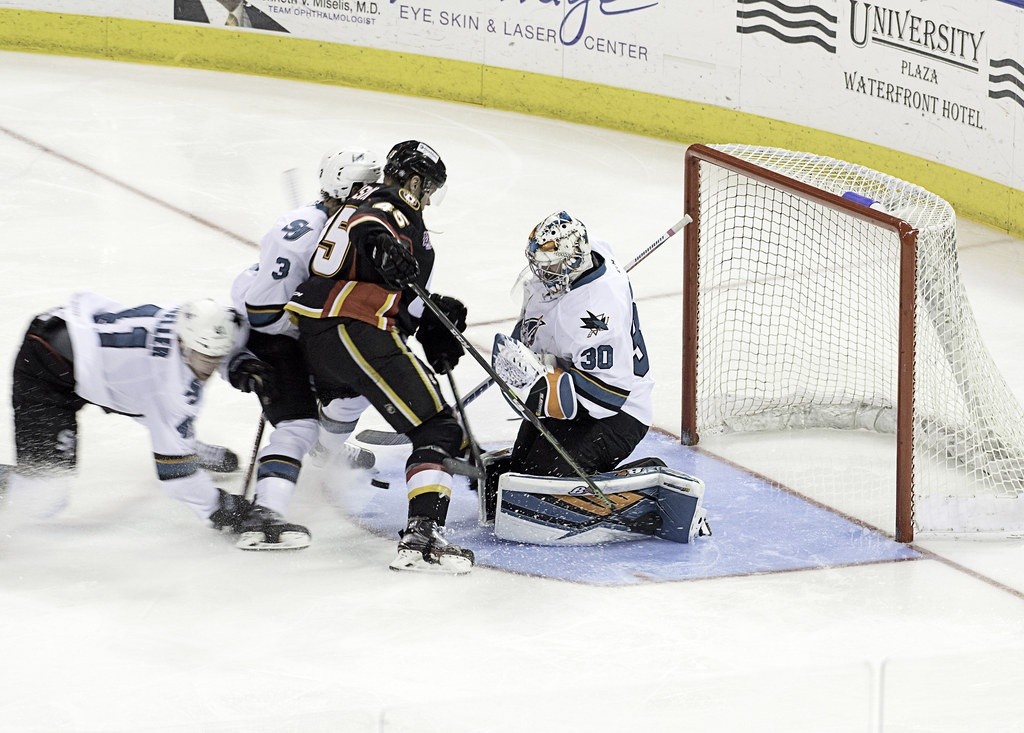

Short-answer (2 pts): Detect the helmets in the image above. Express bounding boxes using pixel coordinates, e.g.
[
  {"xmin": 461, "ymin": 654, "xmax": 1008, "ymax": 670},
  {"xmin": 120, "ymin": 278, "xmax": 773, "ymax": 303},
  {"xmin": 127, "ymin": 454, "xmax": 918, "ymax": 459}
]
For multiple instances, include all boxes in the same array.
[
  {"xmin": 179, "ymin": 297, "xmax": 249, "ymax": 358},
  {"xmin": 525, "ymin": 212, "xmax": 590, "ymax": 292},
  {"xmin": 384, "ymin": 141, "xmax": 448, "ymax": 191},
  {"xmin": 319, "ymin": 148, "xmax": 382, "ymax": 203}
]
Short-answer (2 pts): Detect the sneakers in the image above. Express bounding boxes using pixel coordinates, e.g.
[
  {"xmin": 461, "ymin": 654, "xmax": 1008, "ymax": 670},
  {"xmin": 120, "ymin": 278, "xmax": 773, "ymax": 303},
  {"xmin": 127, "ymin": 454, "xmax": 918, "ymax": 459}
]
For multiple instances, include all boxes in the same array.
[
  {"xmin": 193, "ymin": 439, "xmax": 240, "ymax": 475},
  {"xmin": 238, "ymin": 503, "xmax": 311, "ymax": 552},
  {"xmin": 389, "ymin": 521, "xmax": 474, "ymax": 572},
  {"xmin": 309, "ymin": 445, "xmax": 376, "ymax": 471}
]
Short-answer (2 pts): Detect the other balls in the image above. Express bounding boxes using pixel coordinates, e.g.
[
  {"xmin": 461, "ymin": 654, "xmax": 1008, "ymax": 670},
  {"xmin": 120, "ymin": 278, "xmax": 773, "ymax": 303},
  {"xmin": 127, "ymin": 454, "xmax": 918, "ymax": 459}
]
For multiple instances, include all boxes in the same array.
[{"xmin": 371, "ymin": 478, "xmax": 391, "ymax": 490}]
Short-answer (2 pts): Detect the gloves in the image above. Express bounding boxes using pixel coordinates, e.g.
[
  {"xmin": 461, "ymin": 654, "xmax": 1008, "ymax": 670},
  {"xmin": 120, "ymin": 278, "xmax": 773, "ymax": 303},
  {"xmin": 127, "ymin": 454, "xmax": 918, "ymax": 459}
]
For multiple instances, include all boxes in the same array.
[
  {"xmin": 423, "ymin": 290, "xmax": 467, "ymax": 336},
  {"xmin": 416, "ymin": 324, "xmax": 465, "ymax": 375},
  {"xmin": 210, "ymin": 489, "xmax": 250, "ymax": 529}
]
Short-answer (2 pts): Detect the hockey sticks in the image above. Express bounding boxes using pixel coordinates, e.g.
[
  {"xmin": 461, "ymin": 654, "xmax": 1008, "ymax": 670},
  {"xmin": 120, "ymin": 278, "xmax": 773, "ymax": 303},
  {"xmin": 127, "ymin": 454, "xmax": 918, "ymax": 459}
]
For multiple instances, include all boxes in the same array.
[
  {"xmin": 354, "ymin": 212, "xmax": 695, "ymax": 447},
  {"xmin": 441, "ymin": 357, "xmax": 488, "ymax": 482},
  {"xmin": 405, "ymin": 281, "xmax": 666, "ymax": 536},
  {"xmin": 209, "ymin": 408, "xmax": 266, "ymax": 528}
]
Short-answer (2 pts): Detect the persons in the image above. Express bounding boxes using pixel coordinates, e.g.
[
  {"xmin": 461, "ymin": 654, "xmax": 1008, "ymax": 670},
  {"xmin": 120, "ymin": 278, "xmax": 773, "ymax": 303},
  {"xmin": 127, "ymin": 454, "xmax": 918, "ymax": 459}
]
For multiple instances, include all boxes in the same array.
[
  {"xmin": 231, "ymin": 141, "xmax": 476, "ymax": 559},
  {"xmin": 0, "ymin": 290, "xmax": 252, "ymax": 534},
  {"xmin": 482, "ymin": 211, "xmax": 713, "ymax": 547}
]
